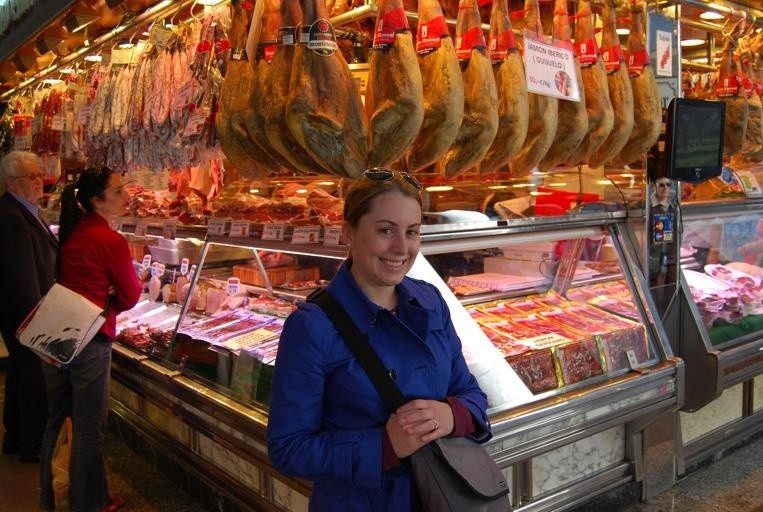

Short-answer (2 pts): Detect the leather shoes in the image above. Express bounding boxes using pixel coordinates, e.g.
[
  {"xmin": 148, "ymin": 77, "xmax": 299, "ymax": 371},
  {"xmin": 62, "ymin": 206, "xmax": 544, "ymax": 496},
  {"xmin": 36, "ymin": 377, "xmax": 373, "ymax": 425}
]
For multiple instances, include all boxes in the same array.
[{"xmin": 98, "ymin": 494, "xmax": 125, "ymax": 512}]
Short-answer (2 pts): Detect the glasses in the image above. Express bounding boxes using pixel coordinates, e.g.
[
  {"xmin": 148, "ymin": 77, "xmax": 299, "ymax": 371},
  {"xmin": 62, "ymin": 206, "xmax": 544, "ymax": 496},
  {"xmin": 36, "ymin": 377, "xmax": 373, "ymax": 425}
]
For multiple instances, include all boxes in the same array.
[
  {"xmin": 8, "ymin": 174, "xmax": 43, "ymax": 180},
  {"xmin": 95, "ymin": 166, "xmax": 110, "ymax": 182},
  {"xmin": 659, "ymin": 183, "xmax": 670, "ymax": 187},
  {"xmin": 358, "ymin": 168, "xmax": 425, "ymax": 193}
]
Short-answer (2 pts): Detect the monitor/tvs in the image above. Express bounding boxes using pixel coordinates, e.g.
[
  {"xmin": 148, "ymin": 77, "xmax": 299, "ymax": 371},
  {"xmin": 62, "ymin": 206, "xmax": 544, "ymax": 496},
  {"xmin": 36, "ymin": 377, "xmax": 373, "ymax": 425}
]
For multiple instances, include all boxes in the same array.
[{"xmin": 664, "ymin": 97, "xmax": 726, "ymax": 181}]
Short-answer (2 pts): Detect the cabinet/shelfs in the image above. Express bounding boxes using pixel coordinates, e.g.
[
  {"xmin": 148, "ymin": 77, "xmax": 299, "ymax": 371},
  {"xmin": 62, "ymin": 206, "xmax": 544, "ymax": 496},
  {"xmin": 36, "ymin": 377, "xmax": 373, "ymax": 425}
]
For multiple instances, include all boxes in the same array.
[{"xmin": 39, "ymin": 194, "xmax": 762, "ymax": 511}]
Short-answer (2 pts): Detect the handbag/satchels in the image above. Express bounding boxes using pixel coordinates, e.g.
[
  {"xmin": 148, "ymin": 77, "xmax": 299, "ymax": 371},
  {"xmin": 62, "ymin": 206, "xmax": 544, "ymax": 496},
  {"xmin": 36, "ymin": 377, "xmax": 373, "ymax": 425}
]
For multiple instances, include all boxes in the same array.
[
  {"xmin": 16, "ymin": 281, "xmax": 109, "ymax": 371},
  {"xmin": 407, "ymin": 434, "xmax": 511, "ymax": 510}
]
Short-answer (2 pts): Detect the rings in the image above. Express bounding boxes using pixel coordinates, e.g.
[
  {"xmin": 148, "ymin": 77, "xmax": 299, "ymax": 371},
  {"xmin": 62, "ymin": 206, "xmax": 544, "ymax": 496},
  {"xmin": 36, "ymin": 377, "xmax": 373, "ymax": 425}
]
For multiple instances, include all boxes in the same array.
[{"xmin": 432, "ymin": 420, "xmax": 437, "ymax": 429}]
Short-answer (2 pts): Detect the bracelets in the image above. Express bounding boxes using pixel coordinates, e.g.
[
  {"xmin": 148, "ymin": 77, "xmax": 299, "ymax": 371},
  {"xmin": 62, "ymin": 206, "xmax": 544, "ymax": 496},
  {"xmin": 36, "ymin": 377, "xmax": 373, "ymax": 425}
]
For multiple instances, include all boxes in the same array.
[{"xmin": 709, "ymin": 248, "xmax": 720, "ymax": 254}]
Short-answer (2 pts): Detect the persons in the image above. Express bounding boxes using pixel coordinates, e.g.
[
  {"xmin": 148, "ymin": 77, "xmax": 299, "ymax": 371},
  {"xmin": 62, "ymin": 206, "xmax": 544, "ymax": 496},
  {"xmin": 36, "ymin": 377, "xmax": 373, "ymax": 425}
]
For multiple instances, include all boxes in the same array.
[
  {"xmin": 30, "ymin": 166, "xmax": 143, "ymax": 510},
  {"xmin": 557, "ymin": 71, "xmax": 571, "ymax": 96},
  {"xmin": 650, "ymin": 178, "xmax": 676, "ymax": 210},
  {"xmin": 706, "ymin": 212, "xmax": 763, "ymax": 269},
  {"xmin": 265, "ymin": 169, "xmax": 493, "ymax": 512},
  {"xmin": 258, "ymin": 249, "xmax": 337, "ymax": 280},
  {"xmin": 680, "ymin": 217, "xmax": 725, "ymax": 274},
  {"xmin": 0, "ymin": 151, "xmax": 61, "ymax": 466}
]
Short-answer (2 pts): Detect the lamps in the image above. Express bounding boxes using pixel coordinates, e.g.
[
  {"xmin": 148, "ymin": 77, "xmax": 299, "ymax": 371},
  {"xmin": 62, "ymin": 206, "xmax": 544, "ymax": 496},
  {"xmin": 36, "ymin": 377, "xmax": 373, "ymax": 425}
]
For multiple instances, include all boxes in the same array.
[{"xmin": 680, "ymin": 24, "xmax": 709, "ymax": 47}]
